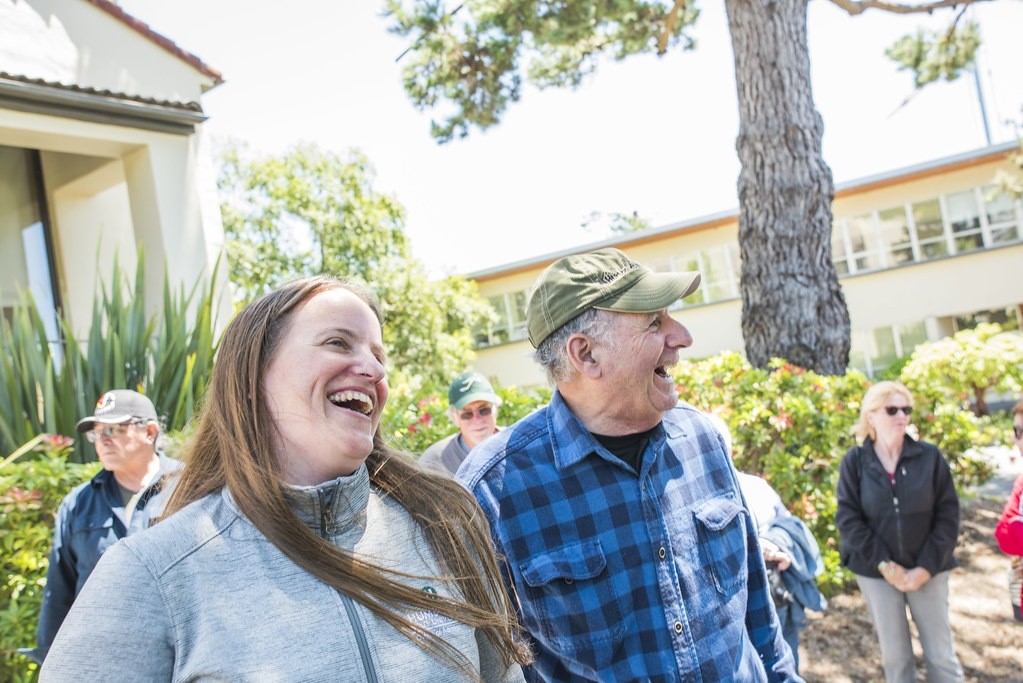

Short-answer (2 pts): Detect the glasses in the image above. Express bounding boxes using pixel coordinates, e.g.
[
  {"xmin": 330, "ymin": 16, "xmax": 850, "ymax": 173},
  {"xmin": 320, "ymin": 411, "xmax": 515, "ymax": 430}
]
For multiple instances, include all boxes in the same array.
[
  {"xmin": 457, "ymin": 404, "xmax": 491, "ymax": 420},
  {"xmin": 84, "ymin": 419, "xmax": 144, "ymax": 443},
  {"xmin": 884, "ymin": 405, "xmax": 913, "ymax": 416},
  {"xmin": 1013, "ymin": 425, "xmax": 1023, "ymax": 439}
]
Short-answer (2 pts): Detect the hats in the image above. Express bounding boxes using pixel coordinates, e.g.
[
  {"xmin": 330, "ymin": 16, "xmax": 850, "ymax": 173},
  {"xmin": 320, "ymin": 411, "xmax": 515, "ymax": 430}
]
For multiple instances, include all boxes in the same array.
[
  {"xmin": 449, "ymin": 372, "xmax": 499, "ymax": 409},
  {"xmin": 74, "ymin": 390, "xmax": 157, "ymax": 433},
  {"xmin": 526, "ymin": 247, "xmax": 701, "ymax": 348}
]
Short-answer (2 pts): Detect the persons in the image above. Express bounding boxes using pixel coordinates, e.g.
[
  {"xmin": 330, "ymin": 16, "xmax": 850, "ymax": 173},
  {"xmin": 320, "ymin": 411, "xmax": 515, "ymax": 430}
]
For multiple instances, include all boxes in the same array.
[
  {"xmin": 17, "ymin": 388, "xmax": 185, "ymax": 666},
  {"xmin": 453, "ymin": 248, "xmax": 805, "ymax": 683},
  {"xmin": 417, "ymin": 371, "xmax": 506, "ymax": 476},
  {"xmin": 39, "ymin": 275, "xmax": 529, "ymax": 683},
  {"xmin": 994, "ymin": 402, "xmax": 1023, "ymax": 618},
  {"xmin": 705, "ymin": 411, "xmax": 799, "ymax": 675},
  {"xmin": 832, "ymin": 381, "xmax": 964, "ymax": 683}
]
